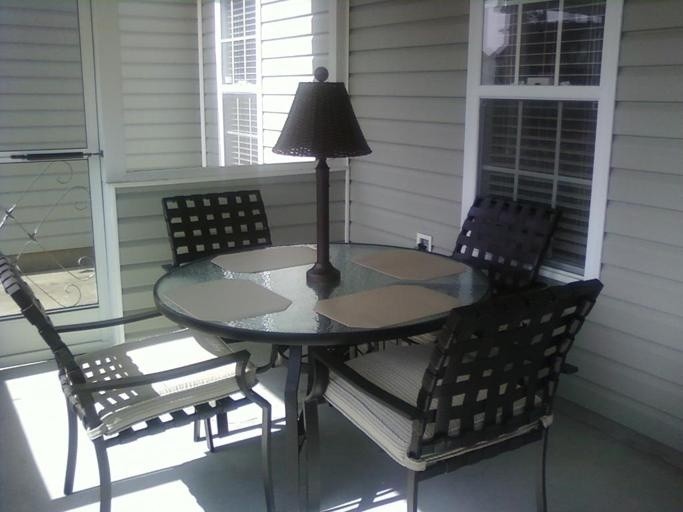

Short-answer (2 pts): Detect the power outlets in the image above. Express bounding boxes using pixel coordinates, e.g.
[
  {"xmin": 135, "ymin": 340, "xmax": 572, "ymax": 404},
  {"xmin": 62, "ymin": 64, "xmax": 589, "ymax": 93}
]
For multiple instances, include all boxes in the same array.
[{"xmin": 415, "ymin": 232, "xmax": 432, "ymax": 252}]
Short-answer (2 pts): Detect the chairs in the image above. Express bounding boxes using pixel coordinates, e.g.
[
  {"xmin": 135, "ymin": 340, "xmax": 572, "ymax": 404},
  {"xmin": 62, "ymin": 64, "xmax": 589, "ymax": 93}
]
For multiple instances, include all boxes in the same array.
[
  {"xmin": 289, "ymin": 275, "xmax": 605, "ymax": 512},
  {"xmin": 153, "ymin": 186, "xmax": 314, "ymax": 439},
  {"xmin": 328, "ymin": 191, "xmax": 567, "ymax": 365},
  {"xmin": 0, "ymin": 246, "xmax": 288, "ymax": 511}
]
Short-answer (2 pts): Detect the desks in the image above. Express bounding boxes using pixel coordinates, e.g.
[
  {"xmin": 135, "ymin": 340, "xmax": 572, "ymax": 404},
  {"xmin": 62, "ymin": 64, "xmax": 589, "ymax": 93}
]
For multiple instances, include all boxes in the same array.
[{"xmin": 147, "ymin": 238, "xmax": 506, "ymax": 511}]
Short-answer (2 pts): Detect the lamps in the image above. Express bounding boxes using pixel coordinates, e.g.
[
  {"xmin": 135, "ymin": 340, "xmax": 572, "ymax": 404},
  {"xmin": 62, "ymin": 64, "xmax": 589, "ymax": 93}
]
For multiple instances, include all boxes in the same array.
[{"xmin": 267, "ymin": 65, "xmax": 373, "ymax": 292}]
